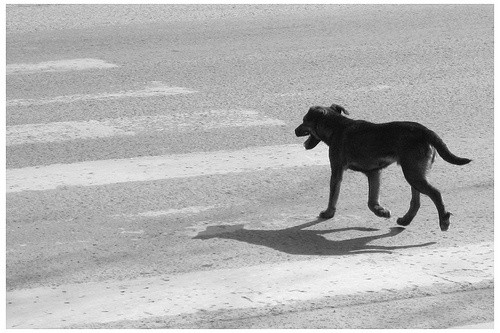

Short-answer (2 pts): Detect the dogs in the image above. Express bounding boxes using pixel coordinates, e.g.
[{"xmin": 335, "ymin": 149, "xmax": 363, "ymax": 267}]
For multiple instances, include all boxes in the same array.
[{"xmin": 294, "ymin": 102, "xmax": 475, "ymax": 231}]
